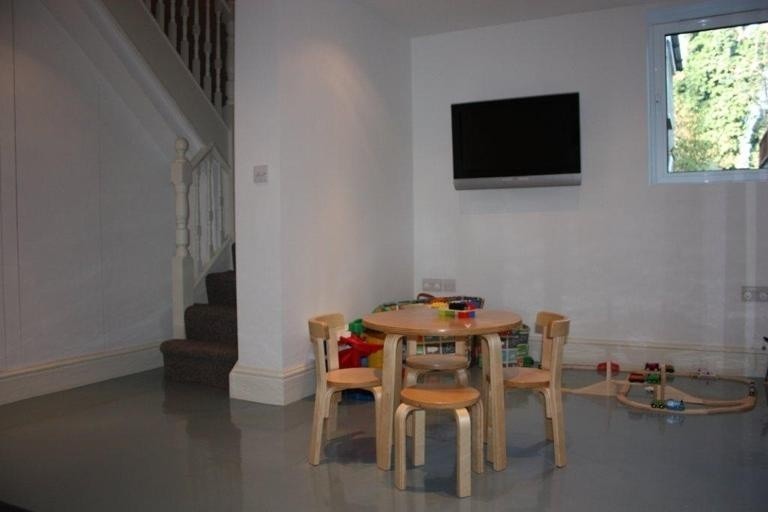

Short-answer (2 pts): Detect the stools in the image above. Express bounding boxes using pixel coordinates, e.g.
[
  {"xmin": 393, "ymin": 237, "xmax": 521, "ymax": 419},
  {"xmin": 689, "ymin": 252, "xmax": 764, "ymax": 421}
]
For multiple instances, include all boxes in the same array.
[
  {"xmin": 394, "ymin": 383, "xmax": 485, "ymax": 497},
  {"xmin": 403, "ymin": 353, "xmax": 471, "ymax": 438}
]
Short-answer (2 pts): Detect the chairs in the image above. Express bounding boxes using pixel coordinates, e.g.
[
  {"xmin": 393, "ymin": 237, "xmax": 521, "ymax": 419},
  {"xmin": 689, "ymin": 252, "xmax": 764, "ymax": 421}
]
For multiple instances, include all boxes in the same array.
[
  {"xmin": 486, "ymin": 311, "xmax": 570, "ymax": 468},
  {"xmin": 308, "ymin": 313, "xmax": 385, "ymax": 465}
]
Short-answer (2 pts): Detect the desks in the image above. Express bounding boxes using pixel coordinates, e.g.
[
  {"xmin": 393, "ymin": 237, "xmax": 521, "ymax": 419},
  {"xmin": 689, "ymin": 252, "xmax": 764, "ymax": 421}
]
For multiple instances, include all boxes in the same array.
[{"xmin": 362, "ymin": 308, "xmax": 523, "ymax": 471}]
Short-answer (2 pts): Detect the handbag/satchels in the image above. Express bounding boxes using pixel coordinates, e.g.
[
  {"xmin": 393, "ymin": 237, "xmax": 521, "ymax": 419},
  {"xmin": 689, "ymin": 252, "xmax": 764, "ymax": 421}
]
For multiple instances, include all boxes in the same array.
[
  {"xmin": 374, "ymin": 293, "xmax": 484, "ymax": 373},
  {"xmin": 476, "ymin": 324, "xmax": 529, "ymax": 368}
]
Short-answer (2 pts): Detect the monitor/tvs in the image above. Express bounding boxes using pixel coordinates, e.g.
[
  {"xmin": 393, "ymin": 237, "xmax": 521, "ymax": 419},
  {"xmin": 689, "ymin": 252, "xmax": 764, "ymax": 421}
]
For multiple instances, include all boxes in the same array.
[{"xmin": 450, "ymin": 91, "xmax": 582, "ymax": 190}]
